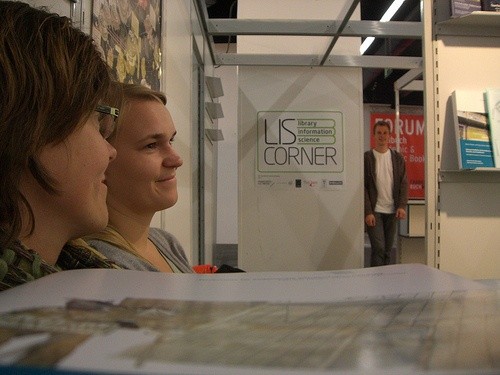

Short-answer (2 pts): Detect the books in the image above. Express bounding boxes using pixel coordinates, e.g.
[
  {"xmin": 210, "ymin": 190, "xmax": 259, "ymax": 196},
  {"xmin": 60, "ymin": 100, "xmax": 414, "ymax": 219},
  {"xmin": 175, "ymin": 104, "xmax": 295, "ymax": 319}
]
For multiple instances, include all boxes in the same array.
[
  {"xmin": 450, "ymin": 0, "xmax": 500, "ymax": 18},
  {"xmin": 450, "ymin": 88, "xmax": 500, "ymax": 171}
]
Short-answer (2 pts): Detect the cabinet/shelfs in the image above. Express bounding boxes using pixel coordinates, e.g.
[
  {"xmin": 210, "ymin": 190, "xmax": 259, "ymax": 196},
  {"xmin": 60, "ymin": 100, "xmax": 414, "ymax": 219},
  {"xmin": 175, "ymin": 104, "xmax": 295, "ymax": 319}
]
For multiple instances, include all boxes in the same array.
[
  {"xmin": 204, "ymin": 76, "xmax": 224, "ymax": 146},
  {"xmin": 423, "ymin": 0, "xmax": 500, "ymax": 279}
]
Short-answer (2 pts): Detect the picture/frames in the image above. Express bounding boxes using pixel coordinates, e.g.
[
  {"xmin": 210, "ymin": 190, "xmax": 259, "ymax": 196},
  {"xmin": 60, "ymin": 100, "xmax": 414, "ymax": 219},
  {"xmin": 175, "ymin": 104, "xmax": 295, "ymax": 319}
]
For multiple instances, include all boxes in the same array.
[{"xmin": 90, "ymin": 0, "xmax": 166, "ymax": 104}]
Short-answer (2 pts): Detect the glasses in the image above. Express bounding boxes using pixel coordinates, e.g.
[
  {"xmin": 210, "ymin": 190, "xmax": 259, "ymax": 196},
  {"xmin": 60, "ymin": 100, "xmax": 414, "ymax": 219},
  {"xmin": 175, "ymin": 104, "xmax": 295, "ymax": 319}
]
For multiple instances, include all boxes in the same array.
[{"xmin": 92, "ymin": 104, "xmax": 120, "ymax": 140}]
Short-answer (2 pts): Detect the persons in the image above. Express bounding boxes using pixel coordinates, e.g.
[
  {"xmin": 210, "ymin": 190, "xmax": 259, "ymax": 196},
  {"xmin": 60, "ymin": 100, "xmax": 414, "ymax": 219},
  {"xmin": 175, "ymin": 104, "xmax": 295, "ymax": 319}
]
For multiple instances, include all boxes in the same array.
[
  {"xmin": 0, "ymin": 0, "xmax": 121, "ymax": 294},
  {"xmin": 365, "ymin": 121, "xmax": 408, "ymax": 266},
  {"xmin": 84, "ymin": 83, "xmax": 200, "ymax": 273}
]
